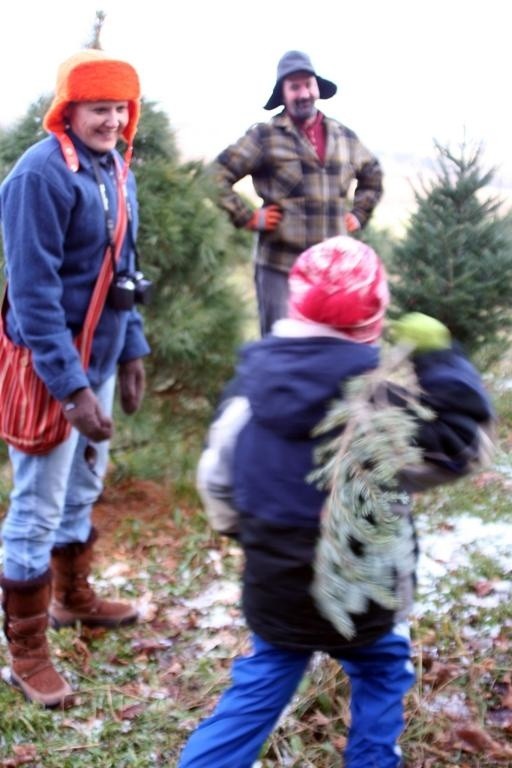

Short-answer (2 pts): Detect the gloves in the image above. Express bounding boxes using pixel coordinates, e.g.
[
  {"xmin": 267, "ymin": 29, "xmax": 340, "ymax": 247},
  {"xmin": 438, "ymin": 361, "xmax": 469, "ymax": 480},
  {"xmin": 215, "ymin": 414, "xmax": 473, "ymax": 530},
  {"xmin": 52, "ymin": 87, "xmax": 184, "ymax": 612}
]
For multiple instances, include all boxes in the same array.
[
  {"xmin": 244, "ymin": 205, "xmax": 282, "ymax": 231},
  {"xmin": 60, "ymin": 386, "xmax": 112, "ymax": 444},
  {"xmin": 343, "ymin": 212, "xmax": 362, "ymax": 233},
  {"xmin": 389, "ymin": 311, "xmax": 452, "ymax": 355},
  {"xmin": 119, "ymin": 358, "xmax": 147, "ymax": 415}
]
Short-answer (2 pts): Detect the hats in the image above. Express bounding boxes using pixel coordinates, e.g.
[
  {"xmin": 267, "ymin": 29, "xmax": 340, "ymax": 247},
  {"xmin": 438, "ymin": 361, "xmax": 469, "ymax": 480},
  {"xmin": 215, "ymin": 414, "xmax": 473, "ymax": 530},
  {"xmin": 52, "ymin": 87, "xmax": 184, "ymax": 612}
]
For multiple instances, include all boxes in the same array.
[
  {"xmin": 287, "ymin": 234, "xmax": 389, "ymax": 344},
  {"xmin": 42, "ymin": 48, "xmax": 141, "ymax": 146},
  {"xmin": 264, "ymin": 50, "xmax": 337, "ymax": 111}
]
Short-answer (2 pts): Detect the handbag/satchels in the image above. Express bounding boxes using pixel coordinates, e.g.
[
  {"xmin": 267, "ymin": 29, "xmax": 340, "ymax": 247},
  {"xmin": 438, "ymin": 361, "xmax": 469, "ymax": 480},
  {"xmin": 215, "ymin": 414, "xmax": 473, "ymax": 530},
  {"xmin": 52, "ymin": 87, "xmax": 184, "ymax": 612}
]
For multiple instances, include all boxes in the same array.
[{"xmin": 0, "ymin": 331, "xmax": 94, "ymax": 455}]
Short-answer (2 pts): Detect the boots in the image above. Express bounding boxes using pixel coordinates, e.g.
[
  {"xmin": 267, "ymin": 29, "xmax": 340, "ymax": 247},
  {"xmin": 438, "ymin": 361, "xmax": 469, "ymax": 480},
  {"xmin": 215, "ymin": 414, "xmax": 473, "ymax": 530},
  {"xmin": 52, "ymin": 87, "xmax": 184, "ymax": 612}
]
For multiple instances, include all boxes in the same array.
[
  {"xmin": 48, "ymin": 526, "xmax": 139, "ymax": 631},
  {"xmin": 0, "ymin": 567, "xmax": 76, "ymax": 710}
]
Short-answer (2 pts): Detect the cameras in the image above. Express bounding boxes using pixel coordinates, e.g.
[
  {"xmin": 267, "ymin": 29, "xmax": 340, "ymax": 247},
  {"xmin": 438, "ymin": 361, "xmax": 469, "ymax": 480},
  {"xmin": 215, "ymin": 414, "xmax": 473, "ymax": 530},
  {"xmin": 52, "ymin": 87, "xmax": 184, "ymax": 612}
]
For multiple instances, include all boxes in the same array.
[{"xmin": 110, "ymin": 270, "xmax": 153, "ymax": 310}]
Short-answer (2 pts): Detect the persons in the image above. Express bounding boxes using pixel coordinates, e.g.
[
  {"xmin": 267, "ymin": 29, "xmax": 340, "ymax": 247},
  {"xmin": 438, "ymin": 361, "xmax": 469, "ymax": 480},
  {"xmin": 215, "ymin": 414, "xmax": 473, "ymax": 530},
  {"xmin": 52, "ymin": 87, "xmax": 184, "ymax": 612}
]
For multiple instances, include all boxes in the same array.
[
  {"xmin": 176, "ymin": 234, "xmax": 491, "ymax": 768},
  {"xmin": 203, "ymin": 50, "xmax": 383, "ymax": 340},
  {"xmin": 0, "ymin": 51, "xmax": 154, "ymax": 711}
]
